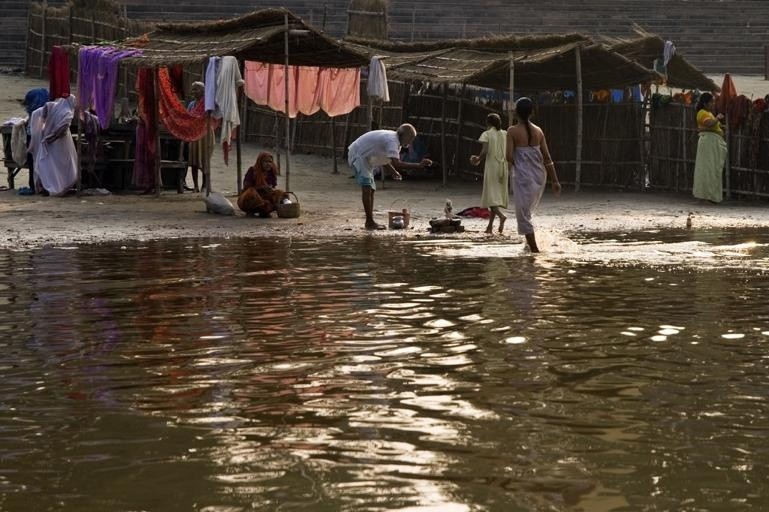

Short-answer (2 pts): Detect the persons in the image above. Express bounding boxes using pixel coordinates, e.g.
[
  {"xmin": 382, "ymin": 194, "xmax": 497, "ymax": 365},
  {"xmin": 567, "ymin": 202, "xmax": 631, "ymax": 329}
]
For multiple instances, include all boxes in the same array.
[
  {"xmin": 236, "ymin": 149, "xmax": 291, "ymax": 218},
  {"xmin": 692, "ymin": 91, "xmax": 730, "ymax": 206},
  {"xmin": 128, "ymin": 112, "xmax": 164, "ymax": 197},
  {"xmin": 472, "ymin": 113, "xmax": 509, "ymax": 233},
  {"xmin": 186, "ymin": 81, "xmax": 214, "ymax": 193},
  {"xmin": 28, "ymin": 94, "xmax": 78, "ymax": 198},
  {"xmin": 347, "ymin": 123, "xmax": 432, "ymax": 231},
  {"xmin": 506, "ymin": 97, "xmax": 562, "ymax": 253}
]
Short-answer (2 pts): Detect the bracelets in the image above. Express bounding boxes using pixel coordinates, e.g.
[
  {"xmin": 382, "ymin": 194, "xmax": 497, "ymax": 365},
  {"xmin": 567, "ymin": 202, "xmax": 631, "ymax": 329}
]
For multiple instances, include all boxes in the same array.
[{"xmin": 543, "ymin": 160, "xmax": 556, "ymax": 169}]
